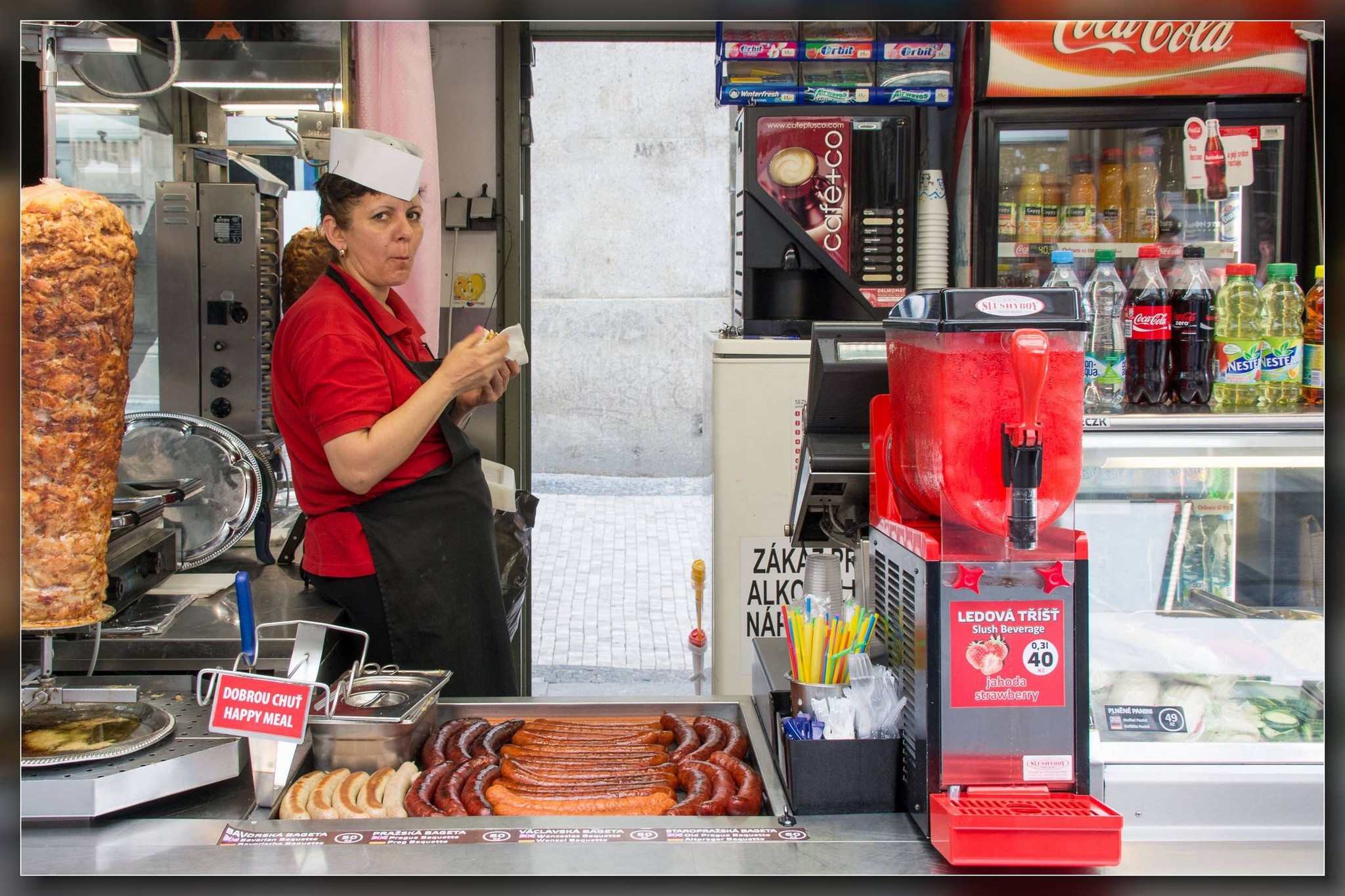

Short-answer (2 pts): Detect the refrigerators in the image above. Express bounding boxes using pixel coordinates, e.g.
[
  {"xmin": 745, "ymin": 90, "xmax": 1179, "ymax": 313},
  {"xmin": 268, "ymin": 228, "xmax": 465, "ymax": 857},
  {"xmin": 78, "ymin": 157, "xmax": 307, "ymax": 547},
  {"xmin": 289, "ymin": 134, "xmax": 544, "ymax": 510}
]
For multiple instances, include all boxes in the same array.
[{"xmin": 952, "ymin": 20, "xmax": 1311, "ymax": 292}]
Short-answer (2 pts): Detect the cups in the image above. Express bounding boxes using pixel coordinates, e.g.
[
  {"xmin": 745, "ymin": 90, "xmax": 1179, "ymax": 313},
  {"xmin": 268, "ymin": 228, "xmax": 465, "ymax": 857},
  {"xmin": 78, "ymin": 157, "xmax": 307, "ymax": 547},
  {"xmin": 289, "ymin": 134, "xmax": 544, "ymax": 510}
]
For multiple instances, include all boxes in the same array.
[
  {"xmin": 802, "ymin": 553, "xmax": 844, "ymax": 618},
  {"xmin": 917, "ymin": 168, "xmax": 950, "ymax": 290},
  {"xmin": 766, "ymin": 146, "xmax": 833, "ymax": 201}
]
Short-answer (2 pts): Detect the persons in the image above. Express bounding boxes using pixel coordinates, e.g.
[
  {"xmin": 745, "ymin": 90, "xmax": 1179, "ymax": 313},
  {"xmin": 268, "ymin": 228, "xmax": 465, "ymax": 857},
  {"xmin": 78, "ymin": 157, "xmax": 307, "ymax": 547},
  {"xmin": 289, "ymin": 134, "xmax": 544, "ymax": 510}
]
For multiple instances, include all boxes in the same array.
[{"xmin": 271, "ymin": 128, "xmax": 521, "ymax": 697}]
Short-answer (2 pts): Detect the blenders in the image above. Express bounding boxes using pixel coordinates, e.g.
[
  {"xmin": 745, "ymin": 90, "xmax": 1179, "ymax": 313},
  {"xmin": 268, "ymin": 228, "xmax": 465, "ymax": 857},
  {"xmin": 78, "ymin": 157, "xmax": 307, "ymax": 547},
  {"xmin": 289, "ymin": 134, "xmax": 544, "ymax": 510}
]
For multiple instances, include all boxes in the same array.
[{"xmin": 867, "ymin": 286, "xmax": 1123, "ymax": 866}]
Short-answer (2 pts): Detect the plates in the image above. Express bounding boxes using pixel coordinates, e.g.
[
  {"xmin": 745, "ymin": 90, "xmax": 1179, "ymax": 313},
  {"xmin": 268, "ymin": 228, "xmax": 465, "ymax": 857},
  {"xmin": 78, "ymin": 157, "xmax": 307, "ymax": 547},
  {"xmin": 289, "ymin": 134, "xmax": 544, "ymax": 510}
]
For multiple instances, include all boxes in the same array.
[{"xmin": 757, "ymin": 152, "xmax": 846, "ymax": 231}]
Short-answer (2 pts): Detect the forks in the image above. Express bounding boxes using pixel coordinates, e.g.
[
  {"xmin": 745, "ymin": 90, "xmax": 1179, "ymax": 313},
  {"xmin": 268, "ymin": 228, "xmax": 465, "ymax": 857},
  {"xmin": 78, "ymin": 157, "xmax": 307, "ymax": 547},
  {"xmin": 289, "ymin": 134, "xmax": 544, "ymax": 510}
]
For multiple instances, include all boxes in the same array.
[{"xmin": 842, "ymin": 653, "xmax": 907, "ymax": 739}]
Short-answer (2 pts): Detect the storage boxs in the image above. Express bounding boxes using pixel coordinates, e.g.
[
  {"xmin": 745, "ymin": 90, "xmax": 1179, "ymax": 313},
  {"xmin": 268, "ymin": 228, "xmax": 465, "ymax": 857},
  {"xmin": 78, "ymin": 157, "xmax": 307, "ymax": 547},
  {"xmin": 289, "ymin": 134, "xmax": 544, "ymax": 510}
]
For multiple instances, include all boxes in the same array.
[{"xmin": 776, "ymin": 712, "xmax": 903, "ymax": 816}]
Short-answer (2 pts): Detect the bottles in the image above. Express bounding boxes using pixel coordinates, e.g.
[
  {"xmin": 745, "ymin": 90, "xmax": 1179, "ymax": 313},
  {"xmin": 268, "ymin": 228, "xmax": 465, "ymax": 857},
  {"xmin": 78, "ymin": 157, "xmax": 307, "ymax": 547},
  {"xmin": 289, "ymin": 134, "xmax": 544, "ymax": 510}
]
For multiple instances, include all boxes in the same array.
[
  {"xmin": 997, "ymin": 245, "xmax": 1324, "ymax": 407},
  {"xmin": 998, "ymin": 142, "xmax": 1185, "ymax": 244}
]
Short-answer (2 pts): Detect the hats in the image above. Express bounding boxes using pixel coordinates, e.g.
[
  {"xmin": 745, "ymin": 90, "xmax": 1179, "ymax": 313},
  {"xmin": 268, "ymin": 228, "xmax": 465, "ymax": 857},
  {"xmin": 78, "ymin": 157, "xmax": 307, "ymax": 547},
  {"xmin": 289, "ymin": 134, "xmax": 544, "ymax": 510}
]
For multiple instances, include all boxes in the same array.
[{"xmin": 329, "ymin": 127, "xmax": 425, "ymax": 201}]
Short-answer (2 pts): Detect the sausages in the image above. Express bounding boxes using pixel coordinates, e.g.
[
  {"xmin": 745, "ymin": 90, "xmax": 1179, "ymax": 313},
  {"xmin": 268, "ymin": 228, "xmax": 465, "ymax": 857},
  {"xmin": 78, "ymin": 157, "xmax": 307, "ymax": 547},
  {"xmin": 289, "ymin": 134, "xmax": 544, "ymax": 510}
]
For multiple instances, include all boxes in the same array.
[
  {"xmin": 659, "ymin": 712, "xmax": 762, "ymax": 816},
  {"xmin": 280, "ymin": 760, "xmax": 420, "ymax": 819},
  {"xmin": 485, "ymin": 718, "xmax": 678, "ymax": 816},
  {"xmin": 403, "ymin": 716, "xmax": 527, "ymax": 816}
]
